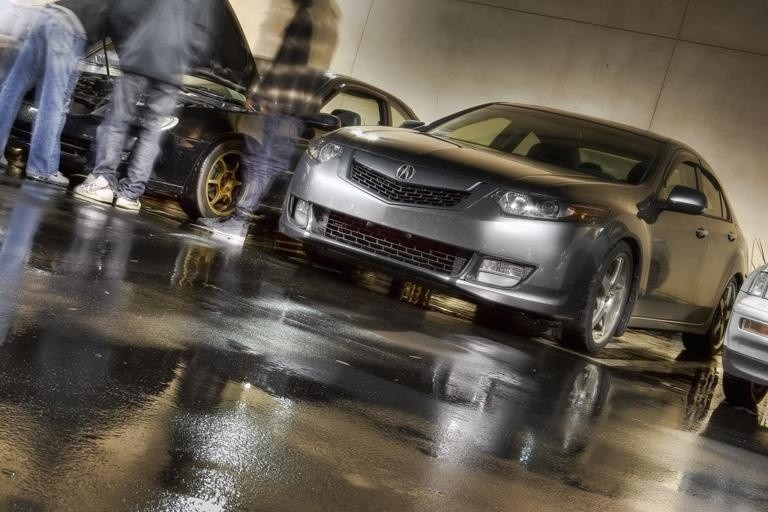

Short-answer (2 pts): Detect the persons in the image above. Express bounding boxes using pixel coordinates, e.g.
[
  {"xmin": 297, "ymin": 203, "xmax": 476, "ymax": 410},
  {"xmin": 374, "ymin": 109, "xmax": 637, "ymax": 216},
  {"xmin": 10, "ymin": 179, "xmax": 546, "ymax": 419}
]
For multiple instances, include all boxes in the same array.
[
  {"xmin": 197, "ymin": 0, "xmax": 342, "ymax": 245},
  {"xmin": 0, "ymin": 0, "xmax": 109, "ymax": 187},
  {"xmin": 72, "ymin": 0, "xmax": 224, "ymax": 210}
]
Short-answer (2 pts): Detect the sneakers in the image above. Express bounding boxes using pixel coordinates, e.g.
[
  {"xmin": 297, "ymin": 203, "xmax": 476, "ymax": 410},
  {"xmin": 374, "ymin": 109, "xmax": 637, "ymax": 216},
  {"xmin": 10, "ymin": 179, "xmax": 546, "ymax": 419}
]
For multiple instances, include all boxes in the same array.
[
  {"xmin": 196, "ymin": 215, "xmax": 252, "ymax": 242},
  {"xmin": 26, "ymin": 168, "xmax": 70, "ymax": 188},
  {"xmin": 115, "ymin": 195, "xmax": 142, "ymax": 211},
  {"xmin": 74, "ymin": 175, "xmax": 114, "ymax": 205},
  {"xmin": 0, "ymin": 156, "xmax": 8, "ymax": 167}
]
